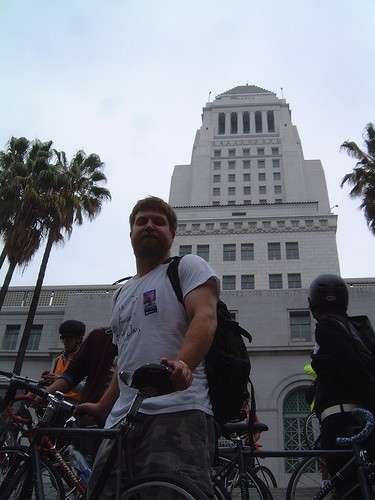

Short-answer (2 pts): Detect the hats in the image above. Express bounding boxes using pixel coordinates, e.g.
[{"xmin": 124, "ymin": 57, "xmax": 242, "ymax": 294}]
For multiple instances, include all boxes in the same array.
[{"xmin": 59, "ymin": 320, "xmax": 85, "ymax": 335}]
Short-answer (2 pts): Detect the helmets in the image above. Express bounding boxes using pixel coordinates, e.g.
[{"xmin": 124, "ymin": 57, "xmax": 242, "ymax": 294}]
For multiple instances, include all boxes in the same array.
[{"xmin": 309, "ymin": 273, "xmax": 349, "ymax": 308}]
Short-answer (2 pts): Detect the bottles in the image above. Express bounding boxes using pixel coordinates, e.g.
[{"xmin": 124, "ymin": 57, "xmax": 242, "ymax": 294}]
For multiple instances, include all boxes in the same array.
[{"xmin": 67, "ymin": 445, "xmax": 92, "ymax": 484}]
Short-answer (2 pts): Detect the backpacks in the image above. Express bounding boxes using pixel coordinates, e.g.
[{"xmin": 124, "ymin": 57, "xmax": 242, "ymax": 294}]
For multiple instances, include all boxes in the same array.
[{"xmin": 113, "ymin": 256, "xmax": 252, "ymax": 421}]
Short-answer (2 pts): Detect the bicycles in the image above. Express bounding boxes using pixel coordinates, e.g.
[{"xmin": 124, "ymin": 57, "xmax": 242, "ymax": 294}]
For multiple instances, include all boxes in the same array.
[{"xmin": 0, "ymin": 362, "xmax": 375, "ymax": 500}]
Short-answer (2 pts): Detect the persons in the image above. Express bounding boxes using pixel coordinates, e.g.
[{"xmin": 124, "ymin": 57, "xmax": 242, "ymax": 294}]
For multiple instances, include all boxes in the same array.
[
  {"xmin": 29, "ymin": 326, "xmax": 118, "ymax": 471},
  {"xmin": 41, "ymin": 320, "xmax": 88, "ymax": 401},
  {"xmin": 73, "ymin": 197, "xmax": 221, "ymax": 500},
  {"xmin": 304, "ymin": 274, "xmax": 375, "ymax": 500}
]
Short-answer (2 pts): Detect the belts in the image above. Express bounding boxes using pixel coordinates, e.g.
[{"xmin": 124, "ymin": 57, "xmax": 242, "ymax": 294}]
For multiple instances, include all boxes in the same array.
[{"xmin": 322, "ymin": 403, "xmax": 356, "ymax": 421}]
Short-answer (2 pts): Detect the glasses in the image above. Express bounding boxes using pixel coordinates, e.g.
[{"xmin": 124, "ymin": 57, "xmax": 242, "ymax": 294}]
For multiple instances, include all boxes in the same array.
[{"xmin": 60, "ymin": 335, "xmax": 80, "ymax": 340}]
